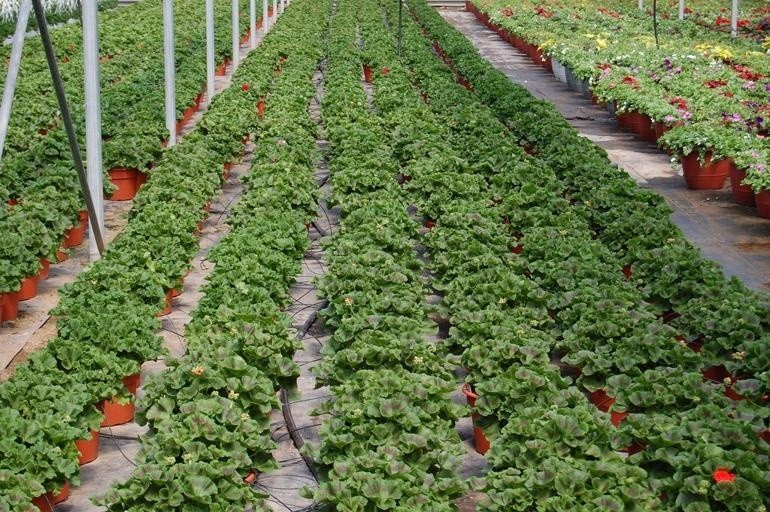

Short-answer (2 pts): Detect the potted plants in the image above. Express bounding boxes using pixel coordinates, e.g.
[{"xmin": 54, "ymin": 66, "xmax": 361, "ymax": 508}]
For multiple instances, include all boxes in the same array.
[{"xmin": 0, "ymin": 0, "xmax": 770, "ymax": 512}]
